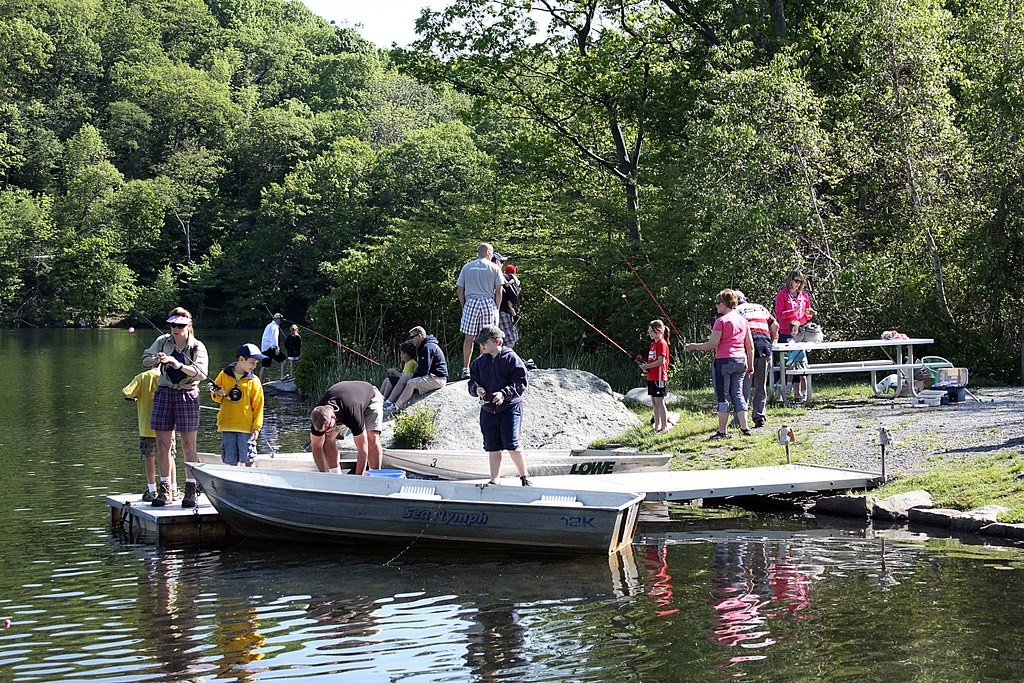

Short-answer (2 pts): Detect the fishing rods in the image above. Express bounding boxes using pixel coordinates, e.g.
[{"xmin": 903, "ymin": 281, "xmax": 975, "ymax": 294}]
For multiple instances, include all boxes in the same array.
[
  {"xmin": 264, "ymin": 304, "xmax": 288, "ymax": 340},
  {"xmin": 283, "ymin": 318, "xmax": 389, "ymax": 370},
  {"xmin": 617, "ymin": 248, "xmax": 701, "ymax": 365},
  {"xmin": 541, "ymin": 287, "xmax": 647, "ymax": 374},
  {"xmin": 97, "ymin": 279, "xmax": 232, "ymax": 401}
]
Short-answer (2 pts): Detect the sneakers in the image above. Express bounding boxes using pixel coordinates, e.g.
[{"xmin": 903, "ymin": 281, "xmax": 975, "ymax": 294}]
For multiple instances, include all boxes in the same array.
[{"xmin": 461, "ymin": 367, "xmax": 471, "ymax": 380}]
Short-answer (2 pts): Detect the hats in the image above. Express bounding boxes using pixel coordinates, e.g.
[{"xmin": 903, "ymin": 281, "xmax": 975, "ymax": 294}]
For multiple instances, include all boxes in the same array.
[
  {"xmin": 471, "ymin": 324, "xmax": 505, "ymax": 344},
  {"xmin": 166, "ymin": 316, "xmax": 192, "ymax": 325},
  {"xmin": 491, "ymin": 252, "xmax": 508, "ymax": 261},
  {"xmin": 273, "ymin": 313, "xmax": 287, "ymax": 321},
  {"xmin": 504, "ymin": 264, "xmax": 517, "ymax": 275},
  {"xmin": 236, "ymin": 343, "xmax": 269, "ymax": 361}
]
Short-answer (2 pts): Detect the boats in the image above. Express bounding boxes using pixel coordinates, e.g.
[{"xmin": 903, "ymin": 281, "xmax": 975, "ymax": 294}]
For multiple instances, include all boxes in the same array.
[
  {"xmin": 385, "ymin": 447, "xmax": 673, "ymax": 479},
  {"xmin": 184, "ymin": 460, "xmax": 646, "ymax": 556}
]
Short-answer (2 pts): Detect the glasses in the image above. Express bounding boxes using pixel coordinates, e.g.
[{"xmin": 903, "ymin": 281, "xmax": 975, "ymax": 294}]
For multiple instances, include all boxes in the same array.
[
  {"xmin": 792, "ymin": 278, "xmax": 802, "ymax": 284},
  {"xmin": 715, "ymin": 301, "xmax": 725, "ymax": 305},
  {"xmin": 409, "ymin": 333, "xmax": 423, "ymax": 339},
  {"xmin": 322, "ymin": 414, "xmax": 333, "ymax": 434},
  {"xmin": 169, "ymin": 323, "xmax": 188, "ymax": 330}
]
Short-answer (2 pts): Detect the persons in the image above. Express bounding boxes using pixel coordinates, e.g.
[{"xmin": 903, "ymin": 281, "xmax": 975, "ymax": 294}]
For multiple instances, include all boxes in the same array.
[
  {"xmin": 284, "ymin": 324, "xmax": 301, "ymax": 378},
  {"xmin": 383, "ymin": 326, "xmax": 448, "ymax": 414},
  {"xmin": 210, "ymin": 343, "xmax": 269, "ymax": 468},
  {"xmin": 639, "ymin": 320, "xmax": 670, "ymax": 434},
  {"xmin": 775, "ymin": 269, "xmax": 814, "ymax": 402},
  {"xmin": 727, "ymin": 291, "xmax": 780, "ymax": 429},
  {"xmin": 491, "ymin": 251, "xmax": 522, "ymax": 349},
  {"xmin": 142, "ymin": 307, "xmax": 209, "ymax": 506},
  {"xmin": 258, "ymin": 313, "xmax": 291, "ymax": 383},
  {"xmin": 309, "ymin": 380, "xmax": 385, "ymax": 475},
  {"xmin": 468, "ymin": 324, "xmax": 534, "ymax": 489},
  {"xmin": 456, "ymin": 243, "xmax": 503, "ymax": 379},
  {"xmin": 123, "ymin": 363, "xmax": 185, "ymax": 501},
  {"xmin": 379, "ymin": 343, "xmax": 419, "ymax": 400},
  {"xmin": 684, "ymin": 289, "xmax": 755, "ymax": 438}
]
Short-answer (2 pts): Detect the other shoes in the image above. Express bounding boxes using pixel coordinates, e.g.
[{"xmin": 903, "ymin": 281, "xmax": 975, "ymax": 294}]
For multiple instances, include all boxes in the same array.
[
  {"xmin": 383, "ymin": 400, "xmax": 393, "ymax": 409},
  {"xmin": 711, "ymin": 431, "xmax": 728, "ymax": 439},
  {"xmin": 512, "ymin": 316, "xmax": 521, "ymax": 326},
  {"xmin": 739, "ymin": 427, "xmax": 752, "ymax": 438},
  {"xmin": 382, "ymin": 403, "xmax": 403, "ymax": 415},
  {"xmin": 753, "ymin": 416, "xmax": 766, "ymax": 428},
  {"xmin": 181, "ymin": 482, "xmax": 201, "ymax": 508},
  {"xmin": 151, "ymin": 482, "xmax": 173, "ymax": 507},
  {"xmin": 171, "ymin": 488, "xmax": 186, "ymax": 501},
  {"xmin": 141, "ymin": 488, "xmax": 158, "ymax": 502}
]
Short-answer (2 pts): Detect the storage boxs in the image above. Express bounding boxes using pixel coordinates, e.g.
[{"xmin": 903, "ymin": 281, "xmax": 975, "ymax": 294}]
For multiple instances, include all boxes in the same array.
[
  {"xmin": 912, "ymin": 389, "xmax": 948, "ymax": 407},
  {"xmin": 933, "ymin": 368, "xmax": 969, "ymax": 402}
]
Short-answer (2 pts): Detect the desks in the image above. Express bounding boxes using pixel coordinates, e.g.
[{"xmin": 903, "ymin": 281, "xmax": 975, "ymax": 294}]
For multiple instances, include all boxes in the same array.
[{"xmin": 765, "ymin": 336, "xmax": 934, "ymax": 407}]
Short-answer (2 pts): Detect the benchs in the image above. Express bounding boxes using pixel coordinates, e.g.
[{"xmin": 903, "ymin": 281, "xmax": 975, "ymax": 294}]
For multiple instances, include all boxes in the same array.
[
  {"xmin": 770, "ymin": 360, "xmax": 952, "ymax": 406},
  {"xmin": 539, "ymin": 495, "xmax": 576, "ymax": 507},
  {"xmin": 400, "ymin": 485, "xmax": 436, "ymax": 500}
]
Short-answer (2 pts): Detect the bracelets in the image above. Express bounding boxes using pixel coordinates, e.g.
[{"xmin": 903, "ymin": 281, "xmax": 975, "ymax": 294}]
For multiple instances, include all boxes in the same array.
[{"xmin": 178, "ymin": 362, "xmax": 183, "ymax": 369}]
